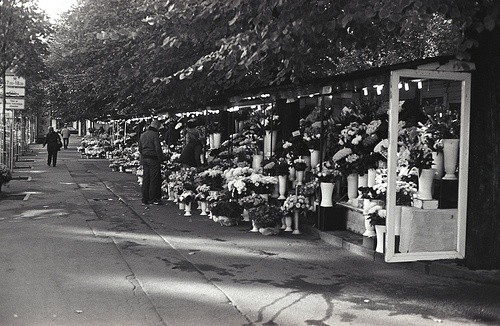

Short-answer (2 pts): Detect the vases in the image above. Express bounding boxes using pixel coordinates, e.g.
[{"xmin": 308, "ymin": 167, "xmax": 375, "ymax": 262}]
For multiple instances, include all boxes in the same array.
[
  {"xmin": 243, "ymin": 209, "xmax": 249, "ymax": 221},
  {"xmin": 200, "ymin": 201, "xmax": 207, "ymax": 215},
  {"xmin": 252, "ymin": 154, "xmax": 261, "ymax": 169},
  {"xmin": 284, "ymin": 216, "xmax": 292, "ymax": 231},
  {"xmin": 363, "ymin": 199, "xmax": 382, "ymax": 236},
  {"xmin": 297, "ymin": 171, "xmax": 303, "ymax": 185},
  {"xmin": 213, "ymin": 132, "xmax": 221, "ymax": 148},
  {"xmin": 184, "ymin": 202, "xmax": 192, "ymax": 216},
  {"xmin": 443, "ymin": 138, "xmax": 459, "ymax": 180},
  {"xmin": 310, "ymin": 150, "xmax": 321, "ymax": 168},
  {"xmin": 430, "ymin": 151, "xmax": 443, "ymax": 180},
  {"xmin": 277, "ymin": 175, "xmax": 286, "ymax": 200},
  {"xmin": 320, "ymin": 181, "xmax": 335, "ymax": 207},
  {"xmin": 264, "ymin": 130, "xmax": 276, "ymax": 156},
  {"xmin": 347, "ymin": 173, "xmax": 357, "ymax": 202},
  {"xmin": 358, "ymin": 174, "xmax": 367, "ymax": 194},
  {"xmin": 210, "ymin": 134, "xmax": 214, "ymax": 149},
  {"xmin": 367, "ymin": 168, "xmax": 375, "ymax": 187},
  {"xmin": 418, "ymin": 169, "xmax": 434, "ymax": 200}
]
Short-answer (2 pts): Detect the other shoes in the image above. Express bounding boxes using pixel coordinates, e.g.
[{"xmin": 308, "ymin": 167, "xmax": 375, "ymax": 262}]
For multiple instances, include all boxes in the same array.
[
  {"xmin": 47, "ymin": 163, "xmax": 50, "ymax": 166},
  {"xmin": 149, "ymin": 200, "xmax": 164, "ymax": 205},
  {"xmin": 142, "ymin": 202, "xmax": 147, "ymax": 206},
  {"xmin": 66, "ymin": 145, "xmax": 67, "ymax": 149},
  {"xmin": 64, "ymin": 147, "xmax": 66, "ymax": 149},
  {"xmin": 53, "ymin": 165, "xmax": 56, "ymax": 167}
]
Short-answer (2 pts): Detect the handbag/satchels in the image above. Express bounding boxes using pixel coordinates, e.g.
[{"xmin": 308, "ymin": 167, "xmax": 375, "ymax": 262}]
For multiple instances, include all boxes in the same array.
[{"xmin": 57, "ymin": 138, "xmax": 62, "ymax": 148}]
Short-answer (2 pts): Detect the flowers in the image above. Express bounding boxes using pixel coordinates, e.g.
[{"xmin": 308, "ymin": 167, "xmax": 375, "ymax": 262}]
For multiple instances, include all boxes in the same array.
[{"xmin": 75, "ymin": 99, "xmax": 458, "ymax": 225}]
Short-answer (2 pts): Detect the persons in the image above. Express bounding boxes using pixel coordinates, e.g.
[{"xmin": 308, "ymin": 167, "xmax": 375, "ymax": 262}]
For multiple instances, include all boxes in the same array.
[
  {"xmin": 178, "ymin": 131, "xmax": 205, "ymax": 168},
  {"xmin": 138, "ymin": 120, "xmax": 166, "ymax": 205},
  {"xmin": 61, "ymin": 125, "xmax": 70, "ymax": 149},
  {"xmin": 42, "ymin": 126, "xmax": 61, "ymax": 167}
]
